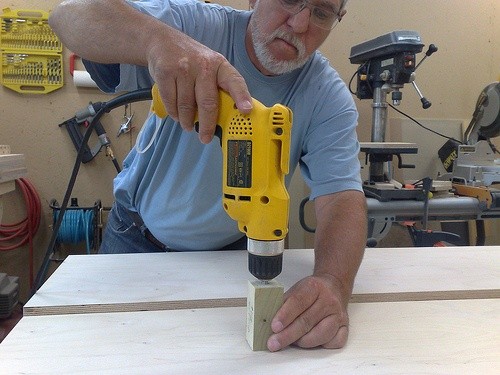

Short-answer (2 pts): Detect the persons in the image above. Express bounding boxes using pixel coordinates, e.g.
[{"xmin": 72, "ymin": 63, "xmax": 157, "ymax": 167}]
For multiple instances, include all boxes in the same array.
[{"xmin": 48, "ymin": 0, "xmax": 369, "ymax": 352}]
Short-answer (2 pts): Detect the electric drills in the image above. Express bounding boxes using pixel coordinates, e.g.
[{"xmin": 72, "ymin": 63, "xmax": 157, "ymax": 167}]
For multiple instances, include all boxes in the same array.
[{"xmin": 149, "ymin": 80, "xmax": 294, "ymax": 285}]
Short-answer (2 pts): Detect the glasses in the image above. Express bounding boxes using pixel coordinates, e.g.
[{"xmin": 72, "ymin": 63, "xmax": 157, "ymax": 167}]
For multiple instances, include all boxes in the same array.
[{"xmin": 280, "ymin": 0, "xmax": 343, "ymax": 30}]
